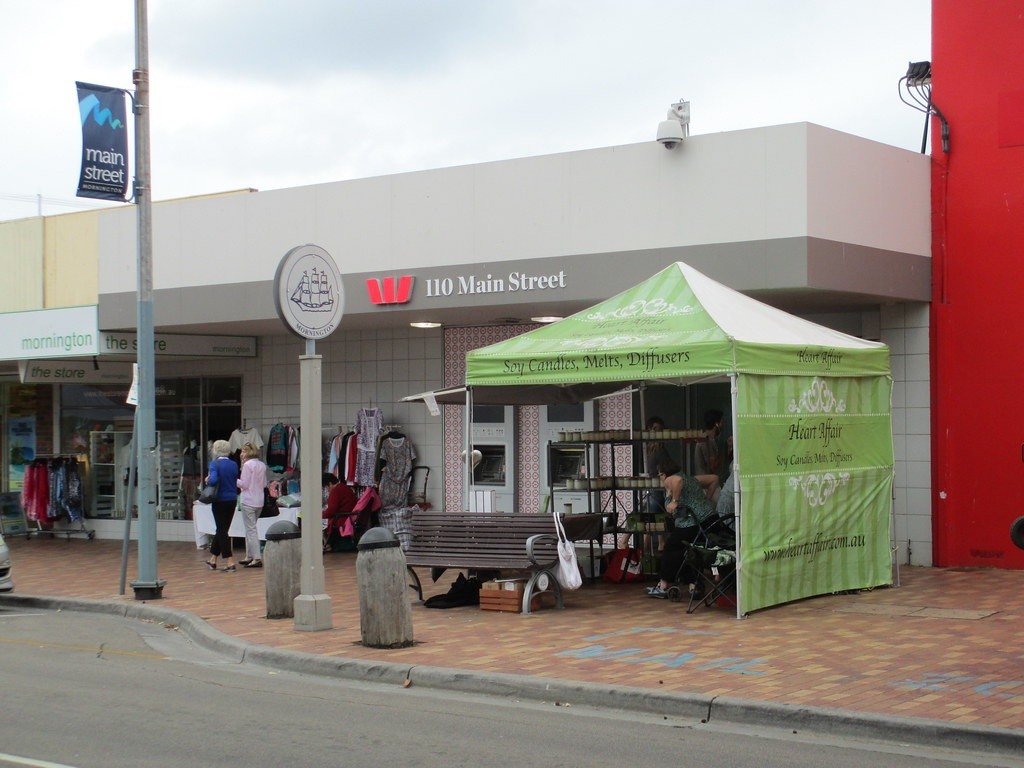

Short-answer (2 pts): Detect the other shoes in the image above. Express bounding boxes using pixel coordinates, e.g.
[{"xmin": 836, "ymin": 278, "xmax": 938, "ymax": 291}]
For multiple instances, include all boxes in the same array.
[
  {"xmin": 245, "ymin": 561, "xmax": 263, "ymax": 568},
  {"xmin": 324, "ymin": 546, "xmax": 333, "ymax": 552},
  {"xmin": 205, "ymin": 559, "xmax": 216, "ymax": 570},
  {"xmin": 648, "ymin": 588, "xmax": 669, "ymax": 599},
  {"xmin": 239, "ymin": 558, "xmax": 253, "ymax": 564},
  {"xmin": 223, "ymin": 565, "xmax": 236, "ymax": 572}
]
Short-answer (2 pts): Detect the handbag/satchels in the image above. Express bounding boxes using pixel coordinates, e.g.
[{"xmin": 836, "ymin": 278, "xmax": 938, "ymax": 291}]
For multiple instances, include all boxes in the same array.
[
  {"xmin": 604, "ymin": 547, "xmax": 645, "ymax": 583},
  {"xmin": 258, "ymin": 487, "xmax": 280, "ymax": 517},
  {"xmin": 199, "ymin": 482, "xmax": 218, "ymax": 505},
  {"xmin": 549, "ymin": 512, "xmax": 583, "ymax": 589}
]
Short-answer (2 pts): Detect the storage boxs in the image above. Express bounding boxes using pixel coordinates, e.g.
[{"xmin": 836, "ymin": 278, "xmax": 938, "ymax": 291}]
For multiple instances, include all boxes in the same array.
[
  {"xmin": 479, "ymin": 589, "xmax": 541, "ymax": 611},
  {"xmin": 482, "ymin": 580, "xmax": 525, "ymax": 590}
]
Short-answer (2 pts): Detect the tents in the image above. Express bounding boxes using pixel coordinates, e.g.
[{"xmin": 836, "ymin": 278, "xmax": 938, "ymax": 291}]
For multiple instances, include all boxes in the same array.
[{"xmin": 463, "ymin": 258, "xmax": 901, "ymax": 619}]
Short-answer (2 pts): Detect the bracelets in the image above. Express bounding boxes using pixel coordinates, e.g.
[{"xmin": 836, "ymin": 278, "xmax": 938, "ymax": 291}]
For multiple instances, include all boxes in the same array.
[{"xmin": 672, "ymin": 499, "xmax": 679, "ymax": 502}]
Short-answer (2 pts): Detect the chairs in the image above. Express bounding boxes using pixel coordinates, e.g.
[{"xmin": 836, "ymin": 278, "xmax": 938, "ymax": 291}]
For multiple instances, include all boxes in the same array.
[
  {"xmin": 674, "ymin": 505, "xmax": 737, "ymax": 614},
  {"xmin": 324, "ymin": 487, "xmax": 382, "ymax": 553}
]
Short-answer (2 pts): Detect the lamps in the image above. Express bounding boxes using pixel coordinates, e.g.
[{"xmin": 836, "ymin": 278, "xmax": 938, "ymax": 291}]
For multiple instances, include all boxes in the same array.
[{"xmin": 93, "ymin": 356, "xmax": 99, "ymax": 370}]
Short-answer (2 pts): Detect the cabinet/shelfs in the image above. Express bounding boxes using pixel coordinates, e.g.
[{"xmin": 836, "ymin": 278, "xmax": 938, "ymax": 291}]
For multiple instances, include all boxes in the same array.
[
  {"xmin": 547, "ymin": 437, "xmax": 709, "ymax": 585},
  {"xmin": 89, "ymin": 431, "xmax": 185, "ymax": 520}
]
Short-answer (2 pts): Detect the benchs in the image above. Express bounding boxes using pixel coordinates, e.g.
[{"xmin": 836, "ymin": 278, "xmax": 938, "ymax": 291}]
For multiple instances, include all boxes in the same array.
[{"xmin": 395, "ymin": 511, "xmax": 566, "ymax": 616}]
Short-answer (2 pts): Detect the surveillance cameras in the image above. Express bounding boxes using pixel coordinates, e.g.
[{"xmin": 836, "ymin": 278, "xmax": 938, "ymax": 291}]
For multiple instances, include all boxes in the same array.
[{"xmin": 656, "ymin": 120, "xmax": 683, "ymax": 150}]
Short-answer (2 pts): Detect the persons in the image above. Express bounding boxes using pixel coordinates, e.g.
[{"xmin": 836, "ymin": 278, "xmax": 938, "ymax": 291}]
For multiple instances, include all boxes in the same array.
[
  {"xmin": 178, "ymin": 440, "xmax": 200, "ymax": 520},
  {"xmin": 236, "ymin": 442, "xmax": 267, "ymax": 567},
  {"xmin": 646, "ymin": 459, "xmax": 719, "ymax": 600},
  {"xmin": 684, "ymin": 409, "xmax": 735, "ymax": 585},
  {"xmin": 205, "ymin": 440, "xmax": 241, "ymax": 572},
  {"xmin": 322, "ymin": 473, "xmax": 358, "ymax": 551},
  {"xmin": 618, "ymin": 418, "xmax": 681, "ymax": 552}
]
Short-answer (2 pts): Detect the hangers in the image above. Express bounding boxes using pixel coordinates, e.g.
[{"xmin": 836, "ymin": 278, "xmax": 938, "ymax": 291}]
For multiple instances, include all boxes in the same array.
[
  {"xmin": 30, "ymin": 454, "xmax": 77, "ymax": 467},
  {"xmin": 274, "ymin": 416, "xmax": 293, "ymax": 429},
  {"xmin": 338, "ymin": 425, "xmax": 398, "ymax": 435},
  {"xmin": 238, "ymin": 423, "xmax": 253, "ymax": 434}
]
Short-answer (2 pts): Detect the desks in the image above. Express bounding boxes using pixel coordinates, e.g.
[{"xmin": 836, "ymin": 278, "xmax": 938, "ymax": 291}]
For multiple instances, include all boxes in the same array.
[
  {"xmin": 192, "ymin": 504, "xmax": 301, "ymax": 553},
  {"xmin": 563, "ymin": 513, "xmax": 604, "ymax": 582}
]
